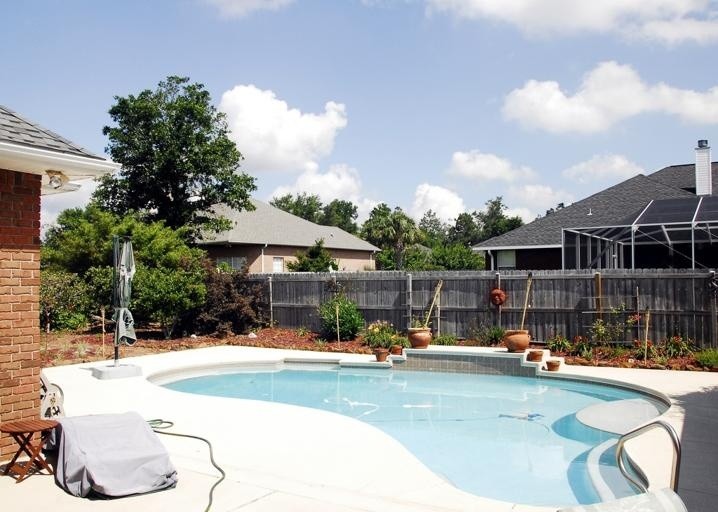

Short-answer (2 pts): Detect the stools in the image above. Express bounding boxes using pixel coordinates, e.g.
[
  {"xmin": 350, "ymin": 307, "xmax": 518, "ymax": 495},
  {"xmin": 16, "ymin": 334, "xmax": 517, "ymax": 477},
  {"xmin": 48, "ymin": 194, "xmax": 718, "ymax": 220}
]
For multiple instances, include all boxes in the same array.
[{"xmin": 0, "ymin": 419, "xmax": 60, "ymax": 483}]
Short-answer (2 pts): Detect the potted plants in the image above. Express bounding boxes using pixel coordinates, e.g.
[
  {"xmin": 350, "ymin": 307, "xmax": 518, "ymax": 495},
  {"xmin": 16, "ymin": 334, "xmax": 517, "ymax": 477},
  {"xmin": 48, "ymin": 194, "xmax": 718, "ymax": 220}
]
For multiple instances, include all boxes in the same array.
[{"xmin": 375, "ymin": 335, "xmax": 405, "ymax": 361}]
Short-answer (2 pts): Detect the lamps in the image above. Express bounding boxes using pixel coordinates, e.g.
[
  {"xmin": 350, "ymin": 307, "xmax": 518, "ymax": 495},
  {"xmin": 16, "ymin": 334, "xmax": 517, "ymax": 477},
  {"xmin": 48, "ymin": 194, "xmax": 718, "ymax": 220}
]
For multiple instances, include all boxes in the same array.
[{"xmin": 44, "ymin": 169, "xmax": 70, "ymax": 189}]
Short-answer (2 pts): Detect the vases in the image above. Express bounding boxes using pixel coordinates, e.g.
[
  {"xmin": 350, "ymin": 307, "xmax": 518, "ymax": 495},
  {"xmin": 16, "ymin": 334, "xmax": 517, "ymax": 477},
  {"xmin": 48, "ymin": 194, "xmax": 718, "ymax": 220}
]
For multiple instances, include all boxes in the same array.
[{"xmin": 407, "ymin": 328, "xmax": 561, "ymax": 372}]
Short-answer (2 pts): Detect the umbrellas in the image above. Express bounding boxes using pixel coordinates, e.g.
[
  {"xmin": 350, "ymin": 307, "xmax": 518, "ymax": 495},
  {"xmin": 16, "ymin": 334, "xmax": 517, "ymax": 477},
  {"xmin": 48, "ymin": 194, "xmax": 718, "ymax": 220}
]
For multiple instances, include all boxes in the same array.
[{"xmin": 110, "ymin": 235, "xmax": 139, "ymax": 362}]
